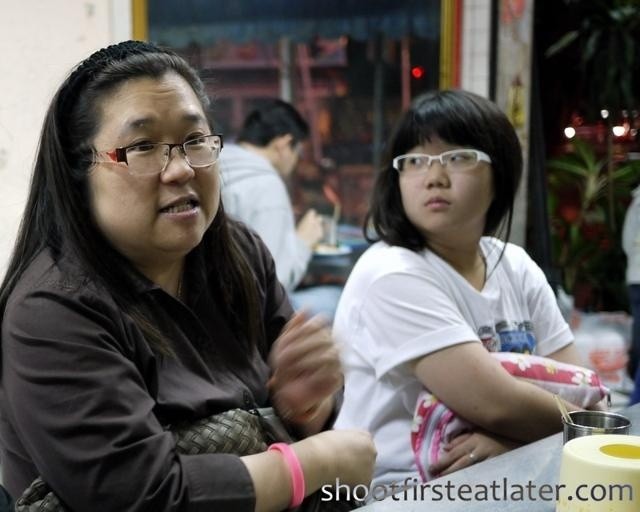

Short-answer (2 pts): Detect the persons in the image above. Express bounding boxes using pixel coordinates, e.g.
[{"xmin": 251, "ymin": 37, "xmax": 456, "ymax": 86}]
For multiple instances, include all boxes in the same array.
[
  {"xmin": 621, "ymin": 185, "xmax": 639, "ymax": 405},
  {"xmin": 2, "ymin": 42, "xmax": 377, "ymax": 512},
  {"xmin": 215, "ymin": 99, "xmax": 325, "ymax": 294},
  {"xmin": 329, "ymin": 88, "xmax": 609, "ymax": 504}
]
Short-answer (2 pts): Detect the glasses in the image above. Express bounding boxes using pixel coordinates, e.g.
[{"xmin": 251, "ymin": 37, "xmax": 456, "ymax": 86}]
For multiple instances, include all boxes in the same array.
[
  {"xmin": 392, "ymin": 146, "xmax": 492, "ymax": 175},
  {"xmin": 74, "ymin": 132, "xmax": 225, "ymax": 178}
]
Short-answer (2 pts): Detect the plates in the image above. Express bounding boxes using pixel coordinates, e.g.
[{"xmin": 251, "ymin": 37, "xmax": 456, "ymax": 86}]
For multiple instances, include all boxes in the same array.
[{"xmin": 312, "ymin": 242, "xmax": 353, "ymax": 257}]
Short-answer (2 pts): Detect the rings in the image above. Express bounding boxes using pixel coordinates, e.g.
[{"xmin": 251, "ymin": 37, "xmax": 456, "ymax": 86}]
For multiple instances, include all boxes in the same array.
[{"xmin": 469, "ymin": 453, "xmax": 478, "ymax": 461}]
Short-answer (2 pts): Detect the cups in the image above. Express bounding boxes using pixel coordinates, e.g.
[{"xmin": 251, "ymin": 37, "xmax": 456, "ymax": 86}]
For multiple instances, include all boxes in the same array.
[{"xmin": 561, "ymin": 411, "xmax": 632, "ymax": 446}]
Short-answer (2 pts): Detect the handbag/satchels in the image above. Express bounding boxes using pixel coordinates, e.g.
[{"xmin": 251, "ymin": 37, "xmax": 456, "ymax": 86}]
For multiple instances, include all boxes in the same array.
[
  {"xmin": 14, "ymin": 404, "xmax": 305, "ymax": 510},
  {"xmin": 407, "ymin": 347, "xmax": 614, "ymax": 484}
]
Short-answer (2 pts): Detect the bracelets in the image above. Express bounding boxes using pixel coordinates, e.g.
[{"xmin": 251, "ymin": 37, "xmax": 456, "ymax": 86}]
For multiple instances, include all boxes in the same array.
[{"xmin": 268, "ymin": 443, "xmax": 305, "ymax": 509}]
